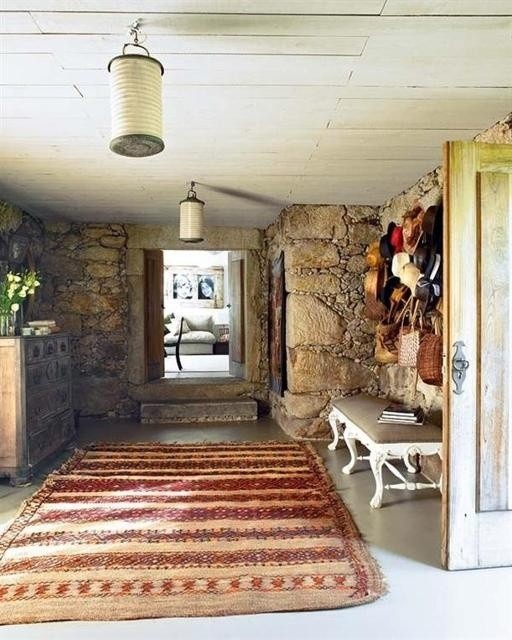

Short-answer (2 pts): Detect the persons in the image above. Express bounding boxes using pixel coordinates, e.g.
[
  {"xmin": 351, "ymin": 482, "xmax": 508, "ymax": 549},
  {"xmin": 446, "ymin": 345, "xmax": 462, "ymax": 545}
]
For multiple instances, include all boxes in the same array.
[
  {"xmin": 173, "ymin": 275, "xmax": 192, "ymax": 299},
  {"xmin": 198, "ymin": 278, "xmax": 214, "ymax": 299}
]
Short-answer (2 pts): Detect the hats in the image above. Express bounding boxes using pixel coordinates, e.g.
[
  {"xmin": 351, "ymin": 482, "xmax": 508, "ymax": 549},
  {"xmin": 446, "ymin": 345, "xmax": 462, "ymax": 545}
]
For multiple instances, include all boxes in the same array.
[{"xmin": 364, "ymin": 206, "xmax": 442, "ymax": 319}]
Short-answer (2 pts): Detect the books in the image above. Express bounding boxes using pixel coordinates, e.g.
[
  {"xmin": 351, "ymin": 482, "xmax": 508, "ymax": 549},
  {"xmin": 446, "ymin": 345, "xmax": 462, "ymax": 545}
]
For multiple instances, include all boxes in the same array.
[{"xmin": 377, "ymin": 404, "xmax": 425, "ymax": 426}]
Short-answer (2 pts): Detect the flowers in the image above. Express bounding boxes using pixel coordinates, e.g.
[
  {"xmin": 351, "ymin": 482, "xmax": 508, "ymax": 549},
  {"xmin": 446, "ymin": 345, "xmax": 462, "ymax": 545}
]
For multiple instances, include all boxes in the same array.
[{"xmin": 0, "ymin": 270, "xmax": 42, "ymax": 325}]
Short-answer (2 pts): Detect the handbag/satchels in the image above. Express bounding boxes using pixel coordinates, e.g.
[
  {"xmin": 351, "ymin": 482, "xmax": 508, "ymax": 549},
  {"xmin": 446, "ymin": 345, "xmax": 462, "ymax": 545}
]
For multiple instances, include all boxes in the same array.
[{"xmin": 375, "ymin": 295, "xmax": 443, "ymax": 386}]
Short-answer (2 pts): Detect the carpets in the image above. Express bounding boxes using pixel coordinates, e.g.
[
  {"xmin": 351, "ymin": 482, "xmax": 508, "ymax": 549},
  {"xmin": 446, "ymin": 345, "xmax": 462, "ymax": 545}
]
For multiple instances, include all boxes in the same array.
[{"xmin": 0, "ymin": 436, "xmax": 389, "ymax": 627}]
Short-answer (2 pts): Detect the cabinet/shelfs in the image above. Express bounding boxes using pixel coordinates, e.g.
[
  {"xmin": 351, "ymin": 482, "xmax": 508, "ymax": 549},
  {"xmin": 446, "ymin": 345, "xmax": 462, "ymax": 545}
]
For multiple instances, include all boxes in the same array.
[{"xmin": 1, "ymin": 331, "xmax": 74, "ymax": 488}]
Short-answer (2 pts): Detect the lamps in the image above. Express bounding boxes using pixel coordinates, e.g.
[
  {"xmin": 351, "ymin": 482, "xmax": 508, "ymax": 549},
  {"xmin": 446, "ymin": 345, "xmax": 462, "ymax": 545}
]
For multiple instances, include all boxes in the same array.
[
  {"xmin": 178, "ymin": 181, "xmax": 206, "ymax": 245},
  {"xmin": 108, "ymin": 18, "xmax": 166, "ymax": 158}
]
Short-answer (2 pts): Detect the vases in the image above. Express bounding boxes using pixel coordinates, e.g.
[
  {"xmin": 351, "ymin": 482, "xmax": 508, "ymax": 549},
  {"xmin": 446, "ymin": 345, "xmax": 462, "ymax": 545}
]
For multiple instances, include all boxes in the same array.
[{"xmin": 1, "ymin": 309, "xmax": 17, "ymax": 336}]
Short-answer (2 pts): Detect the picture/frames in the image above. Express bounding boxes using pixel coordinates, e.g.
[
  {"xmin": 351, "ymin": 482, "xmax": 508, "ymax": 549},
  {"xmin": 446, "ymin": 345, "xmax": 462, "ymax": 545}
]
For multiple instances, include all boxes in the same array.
[{"xmin": 163, "ymin": 265, "xmax": 225, "ymax": 309}]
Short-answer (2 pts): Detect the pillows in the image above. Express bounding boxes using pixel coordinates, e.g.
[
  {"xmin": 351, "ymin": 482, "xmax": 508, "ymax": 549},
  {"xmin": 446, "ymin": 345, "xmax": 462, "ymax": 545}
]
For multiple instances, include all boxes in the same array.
[{"xmin": 164, "ymin": 316, "xmax": 191, "ymax": 335}]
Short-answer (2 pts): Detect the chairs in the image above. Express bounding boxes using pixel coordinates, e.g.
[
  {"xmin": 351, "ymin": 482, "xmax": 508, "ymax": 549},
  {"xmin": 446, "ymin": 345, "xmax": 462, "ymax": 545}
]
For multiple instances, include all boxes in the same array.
[{"xmin": 164, "ymin": 317, "xmax": 185, "ymax": 371}]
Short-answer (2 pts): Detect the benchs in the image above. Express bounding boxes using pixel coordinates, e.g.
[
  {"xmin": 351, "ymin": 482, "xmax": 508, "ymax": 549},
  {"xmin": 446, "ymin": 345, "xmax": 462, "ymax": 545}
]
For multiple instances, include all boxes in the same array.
[{"xmin": 327, "ymin": 397, "xmax": 442, "ymax": 510}]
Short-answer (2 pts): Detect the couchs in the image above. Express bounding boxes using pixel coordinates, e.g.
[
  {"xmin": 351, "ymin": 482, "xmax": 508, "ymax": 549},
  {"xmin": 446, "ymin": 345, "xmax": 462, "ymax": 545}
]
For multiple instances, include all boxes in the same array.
[{"xmin": 164, "ymin": 314, "xmax": 219, "ymax": 354}]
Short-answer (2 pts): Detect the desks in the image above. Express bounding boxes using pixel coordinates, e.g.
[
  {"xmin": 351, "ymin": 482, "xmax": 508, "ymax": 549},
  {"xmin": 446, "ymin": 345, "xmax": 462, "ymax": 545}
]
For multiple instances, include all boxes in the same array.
[{"xmin": 213, "ymin": 342, "xmax": 229, "ymax": 355}]
